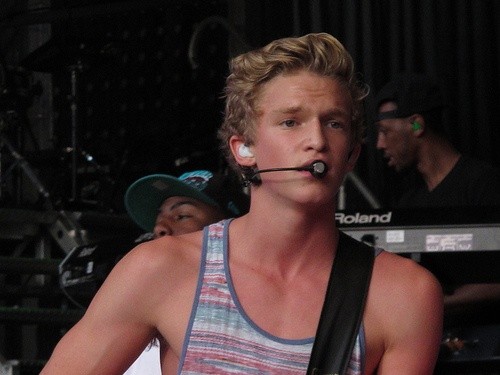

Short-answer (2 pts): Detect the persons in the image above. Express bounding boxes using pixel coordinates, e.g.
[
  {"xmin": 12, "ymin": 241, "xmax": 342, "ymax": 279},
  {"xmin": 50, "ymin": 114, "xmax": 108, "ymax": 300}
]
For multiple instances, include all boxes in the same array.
[
  {"xmin": 124, "ymin": 170, "xmax": 243, "ymax": 240},
  {"xmin": 38, "ymin": 32, "xmax": 444, "ymax": 375},
  {"xmin": 374, "ymin": 88, "xmax": 500, "ymax": 375}
]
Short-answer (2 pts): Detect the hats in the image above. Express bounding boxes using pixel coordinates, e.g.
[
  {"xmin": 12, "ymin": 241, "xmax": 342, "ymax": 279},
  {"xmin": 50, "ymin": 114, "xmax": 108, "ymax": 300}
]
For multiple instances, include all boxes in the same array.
[
  {"xmin": 124, "ymin": 169, "xmax": 246, "ymax": 233},
  {"xmin": 371, "ymin": 73, "xmax": 448, "ymax": 120}
]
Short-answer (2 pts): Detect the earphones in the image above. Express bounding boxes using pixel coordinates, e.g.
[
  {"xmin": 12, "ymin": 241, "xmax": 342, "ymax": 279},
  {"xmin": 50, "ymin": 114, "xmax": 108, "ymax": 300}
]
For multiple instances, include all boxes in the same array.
[
  {"xmin": 412, "ymin": 122, "xmax": 421, "ymax": 132},
  {"xmin": 239, "ymin": 144, "xmax": 252, "ymax": 158}
]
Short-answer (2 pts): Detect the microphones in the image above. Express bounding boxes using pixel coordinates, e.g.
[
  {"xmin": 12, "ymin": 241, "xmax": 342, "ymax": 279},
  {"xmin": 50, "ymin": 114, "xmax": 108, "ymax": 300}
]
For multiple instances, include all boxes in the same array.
[{"xmin": 238, "ymin": 160, "xmax": 329, "ymax": 187}]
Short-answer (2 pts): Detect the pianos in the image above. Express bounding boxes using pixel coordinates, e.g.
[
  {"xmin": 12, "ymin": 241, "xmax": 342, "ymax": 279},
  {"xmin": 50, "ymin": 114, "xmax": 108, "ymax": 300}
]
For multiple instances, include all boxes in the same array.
[{"xmin": 334, "ymin": 202, "xmax": 500, "ymax": 256}]
